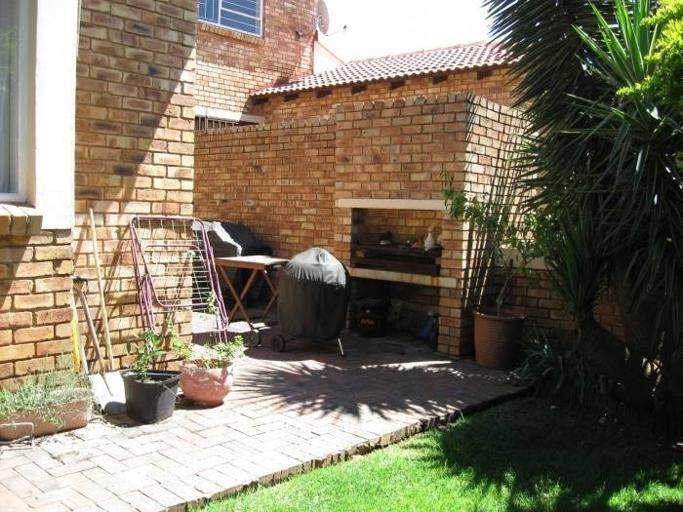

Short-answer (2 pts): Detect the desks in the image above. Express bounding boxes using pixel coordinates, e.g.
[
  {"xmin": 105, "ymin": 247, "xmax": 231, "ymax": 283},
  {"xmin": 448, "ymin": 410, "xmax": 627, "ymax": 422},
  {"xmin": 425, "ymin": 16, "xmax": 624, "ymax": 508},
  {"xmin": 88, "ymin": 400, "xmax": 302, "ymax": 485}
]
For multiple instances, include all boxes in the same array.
[{"xmin": 213, "ymin": 254, "xmax": 290, "ymax": 329}]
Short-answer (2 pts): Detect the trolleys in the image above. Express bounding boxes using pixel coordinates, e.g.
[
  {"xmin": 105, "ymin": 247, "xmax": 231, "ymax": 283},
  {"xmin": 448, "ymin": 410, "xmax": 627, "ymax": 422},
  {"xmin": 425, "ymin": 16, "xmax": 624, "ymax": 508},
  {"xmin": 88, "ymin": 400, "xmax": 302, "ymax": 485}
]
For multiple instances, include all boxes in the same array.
[{"xmin": 211, "ymin": 254, "xmax": 290, "ymax": 347}]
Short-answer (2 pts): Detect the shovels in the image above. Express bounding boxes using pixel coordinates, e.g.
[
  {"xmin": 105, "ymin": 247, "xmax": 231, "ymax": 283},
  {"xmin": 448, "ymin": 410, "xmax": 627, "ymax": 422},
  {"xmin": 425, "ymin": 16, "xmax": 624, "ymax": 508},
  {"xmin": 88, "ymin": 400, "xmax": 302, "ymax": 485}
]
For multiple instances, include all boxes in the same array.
[{"xmin": 73, "ymin": 278, "xmax": 127, "ymax": 415}]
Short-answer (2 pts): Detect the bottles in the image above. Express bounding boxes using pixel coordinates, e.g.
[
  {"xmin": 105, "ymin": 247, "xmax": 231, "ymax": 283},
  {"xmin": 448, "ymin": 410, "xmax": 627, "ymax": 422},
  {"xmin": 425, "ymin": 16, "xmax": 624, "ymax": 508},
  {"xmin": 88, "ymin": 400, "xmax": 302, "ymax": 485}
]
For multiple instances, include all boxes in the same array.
[{"xmin": 423, "ymin": 229, "xmax": 435, "ymax": 253}]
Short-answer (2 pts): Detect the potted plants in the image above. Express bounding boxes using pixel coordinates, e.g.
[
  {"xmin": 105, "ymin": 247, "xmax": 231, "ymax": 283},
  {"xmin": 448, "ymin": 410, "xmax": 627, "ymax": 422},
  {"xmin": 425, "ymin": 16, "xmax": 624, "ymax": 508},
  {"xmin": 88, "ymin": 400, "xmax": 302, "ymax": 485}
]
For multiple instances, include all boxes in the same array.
[
  {"xmin": 436, "ymin": 166, "xmax": 563, "ymax": 371},
  {"xmin": 0, "ymin": 289, "xmax": 244, "ymax": 441}
]
